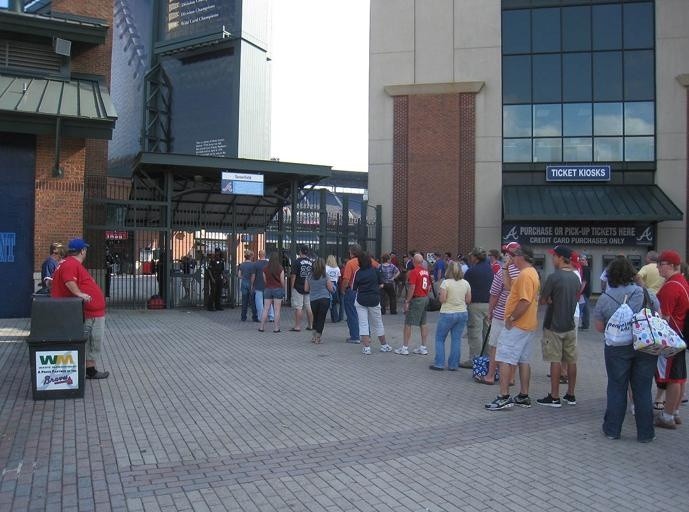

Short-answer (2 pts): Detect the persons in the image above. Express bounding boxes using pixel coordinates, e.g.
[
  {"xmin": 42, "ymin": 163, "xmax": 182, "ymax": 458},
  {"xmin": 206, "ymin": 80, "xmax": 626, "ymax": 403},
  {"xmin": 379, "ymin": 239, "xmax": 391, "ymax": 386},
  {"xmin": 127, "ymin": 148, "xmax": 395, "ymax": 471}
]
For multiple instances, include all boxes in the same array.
[
  {"xmin": 394, "ymin": 253, "xmax": 431, "ymax": 355},
  {"xmin": 378, "ymin": 249, "xmax": 469, "ymax": 315},
  {"xmin": 485, "ymin": 246, "xmax": 689, "ymax": 410},
  {"xmin": 206, "ymin": 247, "xmax": 230, "ymax": 311},
  {"xmin": 50, "ymin": 238, "xmax": 109, "ymax": 380},
  {"xmin": 41, "ymin": 241, "xmax": 65, "ymax": 289},
  {"xmin": 289, "ymin": 244, "xmax": 393, "ymax": 355},
  {"xmin": 177, "ymin": 254, "xmax": 197, "ymax": 299},
  {"xmin": 429, "ymin": 259, "xmax": 471, "ymax": 372},
  {"xmin": 463, "ymin": 241, "xmax": 522, "ymax": 387},
  {"xmin": 105, "ymin": 246, "xmax": 114, "ymax": 298},
  {"xmin": 592, "ymin": 254, "xmax": 663, "ymax": 444},
  {"xmin": 650, "ymin": 249, "xmax": 688, "ymax": 429},
  {"xmin": 237, "ymin": 249, "xmax": 285, "ymax": 333}
]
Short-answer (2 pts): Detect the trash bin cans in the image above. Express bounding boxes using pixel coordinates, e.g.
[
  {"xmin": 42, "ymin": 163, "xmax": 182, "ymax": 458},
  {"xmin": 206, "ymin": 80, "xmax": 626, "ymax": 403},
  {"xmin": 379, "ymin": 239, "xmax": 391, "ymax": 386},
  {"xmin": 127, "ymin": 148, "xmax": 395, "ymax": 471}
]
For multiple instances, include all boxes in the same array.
[{"xmin": 24, "ymin": 296, "xmax": 88, "ymax": 402}]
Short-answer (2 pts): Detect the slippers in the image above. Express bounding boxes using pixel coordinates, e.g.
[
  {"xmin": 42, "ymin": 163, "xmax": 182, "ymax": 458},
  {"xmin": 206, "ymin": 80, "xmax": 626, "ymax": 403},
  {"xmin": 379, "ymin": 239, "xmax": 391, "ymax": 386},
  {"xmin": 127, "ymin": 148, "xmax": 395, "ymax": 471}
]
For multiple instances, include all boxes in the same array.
[
  {"xmin": 289, "ymin": 328, "xmax": 301, "ymax": 331},
  {"xmin": 653, "ymin": 400, "xmax": 666, "ymax": 410}
]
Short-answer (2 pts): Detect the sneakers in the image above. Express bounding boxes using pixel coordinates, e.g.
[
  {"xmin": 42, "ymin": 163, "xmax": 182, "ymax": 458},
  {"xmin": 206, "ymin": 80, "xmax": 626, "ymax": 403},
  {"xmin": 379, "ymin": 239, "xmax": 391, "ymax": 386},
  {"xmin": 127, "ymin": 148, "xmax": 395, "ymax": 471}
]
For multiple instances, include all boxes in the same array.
[
  {"xmin": 360, "ymin": 345, "xmax": 430, "ymax": 355},
  {"xmin": 653, "ymin": 412, "xmax": 682, "ymax": 429},
  {"xmin": 478, "ymin": 393, "xmax": 580, "ymax": 412},
  {"xmin": 86, "ymin": 371, "xmax": 110, "ymax": 379},
  {"xmin": 429, "ymin": 365, "xmax": 443, "ymax": 370},
  {"xmin": 312, "ymin": 336, "xmax": 323, "ymax": 344}
]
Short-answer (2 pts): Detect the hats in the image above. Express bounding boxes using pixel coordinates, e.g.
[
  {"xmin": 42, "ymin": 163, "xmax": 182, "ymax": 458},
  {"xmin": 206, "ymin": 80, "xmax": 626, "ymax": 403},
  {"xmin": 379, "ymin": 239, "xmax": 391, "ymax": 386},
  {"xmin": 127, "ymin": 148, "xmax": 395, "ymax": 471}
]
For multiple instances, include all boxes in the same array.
[
  {"xmin": 68, "ymin": 239, "xmax": 90, "ymax": 252},
  {"xmin": 467, "ymin": 247, "xmax": 487, "ymax": 259},
  {"xmin": 502, "ymin": 242, "xmax": 534, "ymax": 259},
  {"xmin": 546, "ymin": 245, "xmax": 582, "ymax": 269},
  {"xmin": 647, "ymin": 249, "xmax": 681, "ymax": 265}
]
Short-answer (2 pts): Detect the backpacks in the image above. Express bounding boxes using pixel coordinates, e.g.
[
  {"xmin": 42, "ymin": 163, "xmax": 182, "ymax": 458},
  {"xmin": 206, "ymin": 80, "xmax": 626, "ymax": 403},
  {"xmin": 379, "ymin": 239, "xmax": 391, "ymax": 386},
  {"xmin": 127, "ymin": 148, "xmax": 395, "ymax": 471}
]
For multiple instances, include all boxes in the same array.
[{"xmin": 603, "ymin": 286, "xmax": 634, "ymax": 347}]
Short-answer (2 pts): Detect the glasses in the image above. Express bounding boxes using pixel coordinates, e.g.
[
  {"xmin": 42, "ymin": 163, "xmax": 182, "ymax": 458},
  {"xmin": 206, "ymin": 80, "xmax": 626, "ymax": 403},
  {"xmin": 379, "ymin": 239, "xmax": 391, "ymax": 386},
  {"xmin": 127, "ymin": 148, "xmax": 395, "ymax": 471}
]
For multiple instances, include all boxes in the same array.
[{"xmin": 50, "ymin": 251, "xmax": 59, "ymax": 256}]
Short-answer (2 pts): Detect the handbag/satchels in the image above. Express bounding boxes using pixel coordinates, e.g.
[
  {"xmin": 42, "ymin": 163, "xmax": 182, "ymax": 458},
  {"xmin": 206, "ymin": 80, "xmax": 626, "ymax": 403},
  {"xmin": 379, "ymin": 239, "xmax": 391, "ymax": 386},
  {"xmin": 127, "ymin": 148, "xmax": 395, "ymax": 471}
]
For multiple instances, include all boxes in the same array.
[
  {"xmin": 426, "ymin": 298, "xmax": 441, "ymax": 311},
  {"xmin": 632, "ymin": 307, "xmax": 687, "ymax": 359},
  {"xmin": 472, "ymin": 355, "xmax": 500, "ymax": 380}
]
